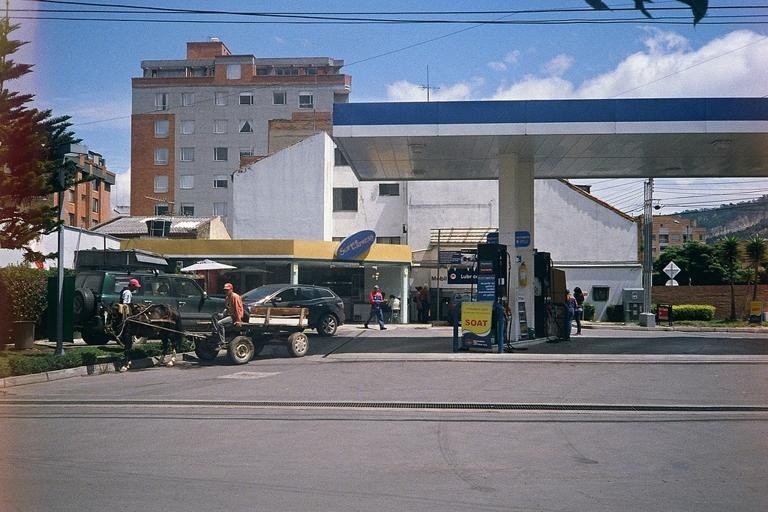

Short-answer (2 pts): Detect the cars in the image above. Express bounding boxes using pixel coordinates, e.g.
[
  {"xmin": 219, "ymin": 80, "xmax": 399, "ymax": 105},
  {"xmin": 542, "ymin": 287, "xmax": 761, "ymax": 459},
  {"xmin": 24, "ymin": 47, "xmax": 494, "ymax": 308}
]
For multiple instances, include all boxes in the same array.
[{"xmin": 239, "ymin": 284, "xmax": 346, "ymax": 338}]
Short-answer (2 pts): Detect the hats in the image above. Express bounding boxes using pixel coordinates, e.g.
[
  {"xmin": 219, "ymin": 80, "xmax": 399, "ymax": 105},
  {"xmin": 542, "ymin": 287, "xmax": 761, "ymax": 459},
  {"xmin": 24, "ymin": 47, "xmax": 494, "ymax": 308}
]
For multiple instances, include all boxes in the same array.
[
  {"xmin": 224, "ymin": 283, "xmax": 233, "ymax": 290},
  {"xmin": 130, "ymin": 279, "xmax": 141, "ymax": 287}
]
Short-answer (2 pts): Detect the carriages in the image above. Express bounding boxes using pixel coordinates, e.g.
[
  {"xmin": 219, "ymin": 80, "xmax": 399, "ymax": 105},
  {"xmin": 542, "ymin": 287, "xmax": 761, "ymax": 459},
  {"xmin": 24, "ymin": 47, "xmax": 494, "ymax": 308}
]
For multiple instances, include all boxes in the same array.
[{"xmin": 98, "ymin": 300, "xmax": 311, "ymax": 365}]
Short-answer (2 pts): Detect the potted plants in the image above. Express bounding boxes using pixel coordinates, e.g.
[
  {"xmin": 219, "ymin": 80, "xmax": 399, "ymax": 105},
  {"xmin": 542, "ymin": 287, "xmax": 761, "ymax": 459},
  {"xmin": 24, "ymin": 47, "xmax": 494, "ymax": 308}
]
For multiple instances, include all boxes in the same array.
[{"xmin": 0, "ymin": 260, "xmax": 50, "ymax": 350}]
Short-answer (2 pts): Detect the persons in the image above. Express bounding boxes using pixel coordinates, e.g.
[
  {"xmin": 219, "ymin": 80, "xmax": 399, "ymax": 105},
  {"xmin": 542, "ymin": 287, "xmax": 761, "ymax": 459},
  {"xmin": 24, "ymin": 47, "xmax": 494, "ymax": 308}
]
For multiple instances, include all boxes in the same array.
[
  {"xmin": 119, "ymin": 278, "xmax": 142, "ymax": 303},
  {"xmin": 574, "ymin": 287, "xmax": 585, "ymax": 334},
  {"xmin": 214, "ymin": 282, "xmax": 245, "ymax": 351},
  {"xmin": 565, "ymin": 289, "xmax": 582, "ymax": 334},
  {"xmin": 380, "ymin": 283, "xmax": 432, "ymax": 324},
  {"xmin": 364, "ymin": 286, "xmax": 386, "ymax": 330}
]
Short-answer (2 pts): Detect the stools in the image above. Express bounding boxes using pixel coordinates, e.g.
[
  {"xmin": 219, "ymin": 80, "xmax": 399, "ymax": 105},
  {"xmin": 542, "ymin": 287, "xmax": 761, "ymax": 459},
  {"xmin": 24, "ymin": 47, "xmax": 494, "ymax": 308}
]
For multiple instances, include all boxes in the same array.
[{"xmin": 391, "ymin": 308, "xmax": 401, "ymax": 324}]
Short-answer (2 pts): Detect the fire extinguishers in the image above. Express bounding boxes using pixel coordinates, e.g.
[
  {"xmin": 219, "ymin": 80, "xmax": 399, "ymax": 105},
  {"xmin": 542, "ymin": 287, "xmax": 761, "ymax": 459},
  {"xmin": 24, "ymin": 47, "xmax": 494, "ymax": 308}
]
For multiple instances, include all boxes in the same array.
[{"xmin": 519, "ymin": 261, "xmax": 528, "ymax": 286}]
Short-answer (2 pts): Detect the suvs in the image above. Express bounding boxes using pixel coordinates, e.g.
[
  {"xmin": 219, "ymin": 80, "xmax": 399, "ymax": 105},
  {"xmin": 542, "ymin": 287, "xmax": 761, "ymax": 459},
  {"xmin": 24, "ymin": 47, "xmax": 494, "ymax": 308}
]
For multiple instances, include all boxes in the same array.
[
  {"xmin": 71, "ymin": 270, "xmax": 226, "ymax": 345},
  {"xmin": 444, "ymin": 293, "xmax": 477, "ymax": 325}
]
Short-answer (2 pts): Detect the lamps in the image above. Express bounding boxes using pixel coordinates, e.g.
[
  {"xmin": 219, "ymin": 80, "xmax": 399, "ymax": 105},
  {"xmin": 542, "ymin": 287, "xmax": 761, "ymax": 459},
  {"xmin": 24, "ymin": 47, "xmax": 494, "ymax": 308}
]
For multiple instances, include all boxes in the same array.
[{"xmin": 370, "ymin": 265, "xmax": 381, "ymax": 281}]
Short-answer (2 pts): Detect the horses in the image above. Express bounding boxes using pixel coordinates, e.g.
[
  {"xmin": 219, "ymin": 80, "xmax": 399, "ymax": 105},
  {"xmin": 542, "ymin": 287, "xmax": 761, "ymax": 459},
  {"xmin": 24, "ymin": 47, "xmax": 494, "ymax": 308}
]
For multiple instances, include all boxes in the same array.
[{"xmin": 100, "ymin": 299, "xmax": 184, "ymax": 372}]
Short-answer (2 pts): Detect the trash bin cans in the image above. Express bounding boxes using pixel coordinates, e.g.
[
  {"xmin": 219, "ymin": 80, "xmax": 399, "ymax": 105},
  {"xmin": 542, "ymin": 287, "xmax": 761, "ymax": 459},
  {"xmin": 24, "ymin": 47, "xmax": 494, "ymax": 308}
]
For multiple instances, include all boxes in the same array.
[{"xmin": 13, "ymin": 321, "xmax": 37, "ymax": 349}]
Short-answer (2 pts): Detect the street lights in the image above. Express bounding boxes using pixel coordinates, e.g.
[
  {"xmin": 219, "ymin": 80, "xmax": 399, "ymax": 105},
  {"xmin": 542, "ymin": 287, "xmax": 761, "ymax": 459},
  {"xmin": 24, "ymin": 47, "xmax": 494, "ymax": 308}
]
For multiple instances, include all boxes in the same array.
[
  {"xmin": 55, "ymin": 144, "xmax": 89, "ymax": 356},
  {"xmin": 674, "ymin": 220, "xmax": 692, "ymax": 287}
]
